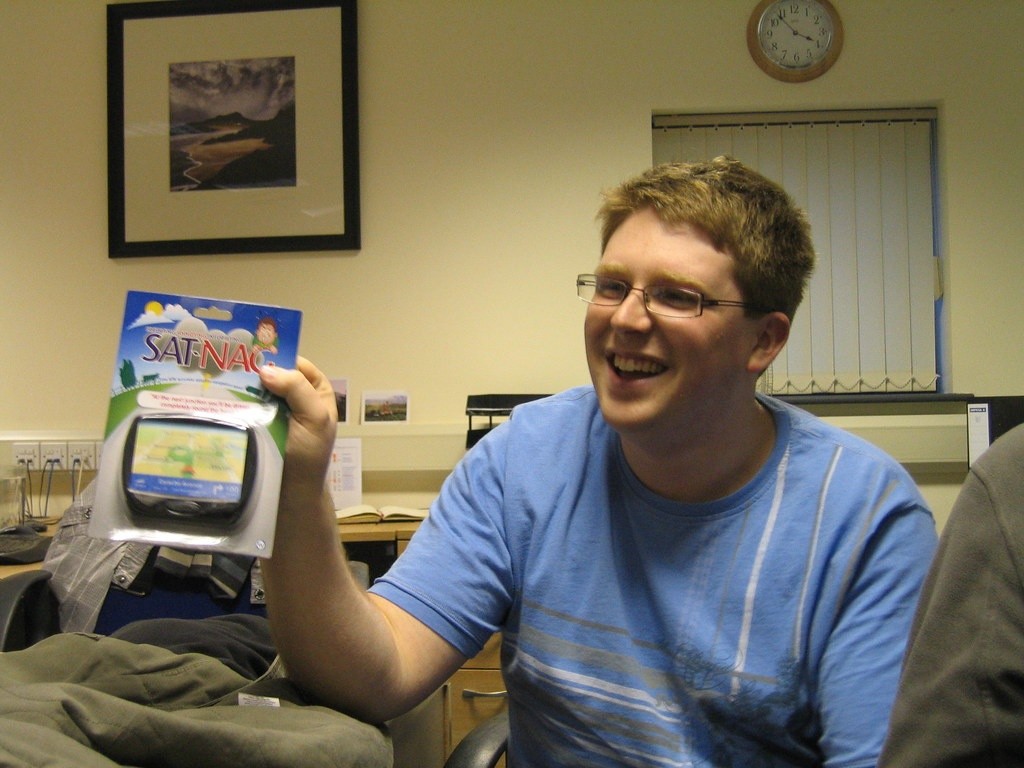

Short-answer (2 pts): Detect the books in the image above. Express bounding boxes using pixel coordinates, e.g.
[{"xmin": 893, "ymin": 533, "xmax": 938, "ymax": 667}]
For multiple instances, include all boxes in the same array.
[{"xmin": 336, "ymin": 506, "xmax": 426, "ymax": 524}]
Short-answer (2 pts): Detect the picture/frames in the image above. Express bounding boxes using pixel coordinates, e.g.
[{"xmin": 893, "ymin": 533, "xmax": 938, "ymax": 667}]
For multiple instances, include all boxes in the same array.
[{"xmin": 107, "ymin": 0, "xmax": 360, "ymax": 259}]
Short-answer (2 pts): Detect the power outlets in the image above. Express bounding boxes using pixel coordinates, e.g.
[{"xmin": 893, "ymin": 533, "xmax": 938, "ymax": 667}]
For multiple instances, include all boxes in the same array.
[
  {"xmin": 39, "ymin": 443, "xmax": 67, "ymax": 471},
  {"xmin": 95, "ymin": 442, "xmax": 105, "ymax": 470},
  {"xmin": 11, "ymin": 443, "xmax": 40, "ymax": 471},
  {"xmin": 67, "ymin": 442, "xmax": 96, "ymax": 471}
]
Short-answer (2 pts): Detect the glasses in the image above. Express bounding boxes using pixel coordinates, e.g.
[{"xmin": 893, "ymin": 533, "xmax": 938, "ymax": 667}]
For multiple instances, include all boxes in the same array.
[{"xmin": 575, "ymin": 274, "xmax": 766, "ymax": 318}]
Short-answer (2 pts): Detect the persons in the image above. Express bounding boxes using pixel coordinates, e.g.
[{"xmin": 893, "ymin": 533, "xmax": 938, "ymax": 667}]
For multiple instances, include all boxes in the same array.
[
  {"xmin": 260, "ymin": 157, "xmax": 939, "ymax": 768},
  {"xmin": 877, "ymin": 422, "xmax": 1024, "ymax": 768}
]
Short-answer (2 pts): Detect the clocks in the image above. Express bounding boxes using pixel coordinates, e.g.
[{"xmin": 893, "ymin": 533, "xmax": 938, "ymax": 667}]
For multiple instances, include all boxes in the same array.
[{"xmin": 746, "ymin": 0, "xmax": 843, "ymax": 83}]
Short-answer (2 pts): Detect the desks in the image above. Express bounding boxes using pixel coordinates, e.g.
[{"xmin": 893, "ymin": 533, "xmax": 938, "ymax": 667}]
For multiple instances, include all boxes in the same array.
[{"xmin": 2, "ymin": 518, "xmax": 512, "ymax": 767}]
